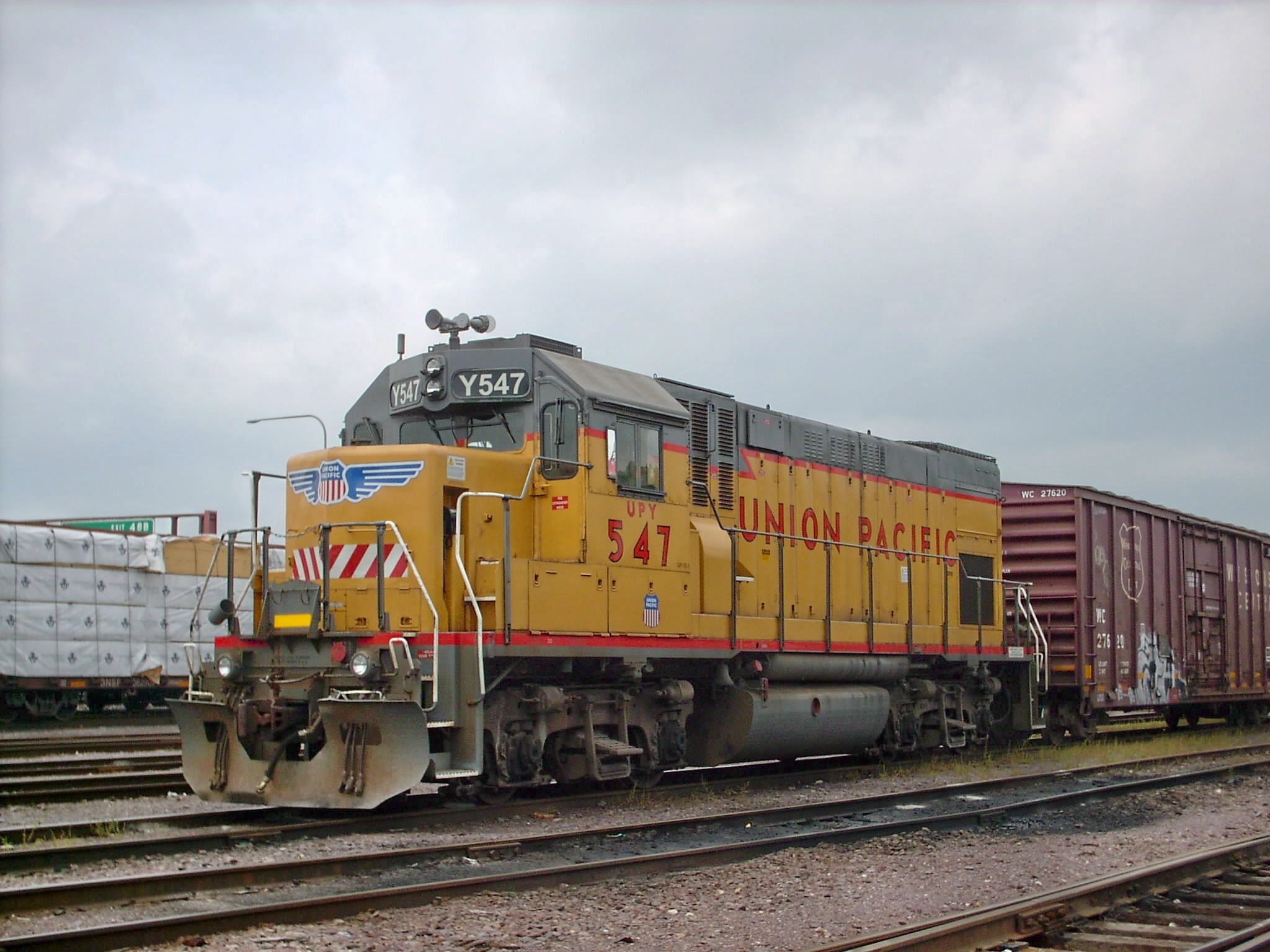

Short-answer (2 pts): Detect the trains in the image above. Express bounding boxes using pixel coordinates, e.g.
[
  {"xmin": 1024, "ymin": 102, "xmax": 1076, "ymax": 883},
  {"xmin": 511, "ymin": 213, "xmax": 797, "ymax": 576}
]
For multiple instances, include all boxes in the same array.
[{"xmin": 165, "ymin": 308, "xmax": 1270, "ymax": 810}]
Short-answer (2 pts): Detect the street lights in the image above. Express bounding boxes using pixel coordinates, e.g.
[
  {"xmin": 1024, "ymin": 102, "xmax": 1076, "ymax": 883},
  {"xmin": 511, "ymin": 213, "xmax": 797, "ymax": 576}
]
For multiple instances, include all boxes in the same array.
[{"xmin": 245, "ymin": 414, "xmax": 328, "ymax": 450}]
